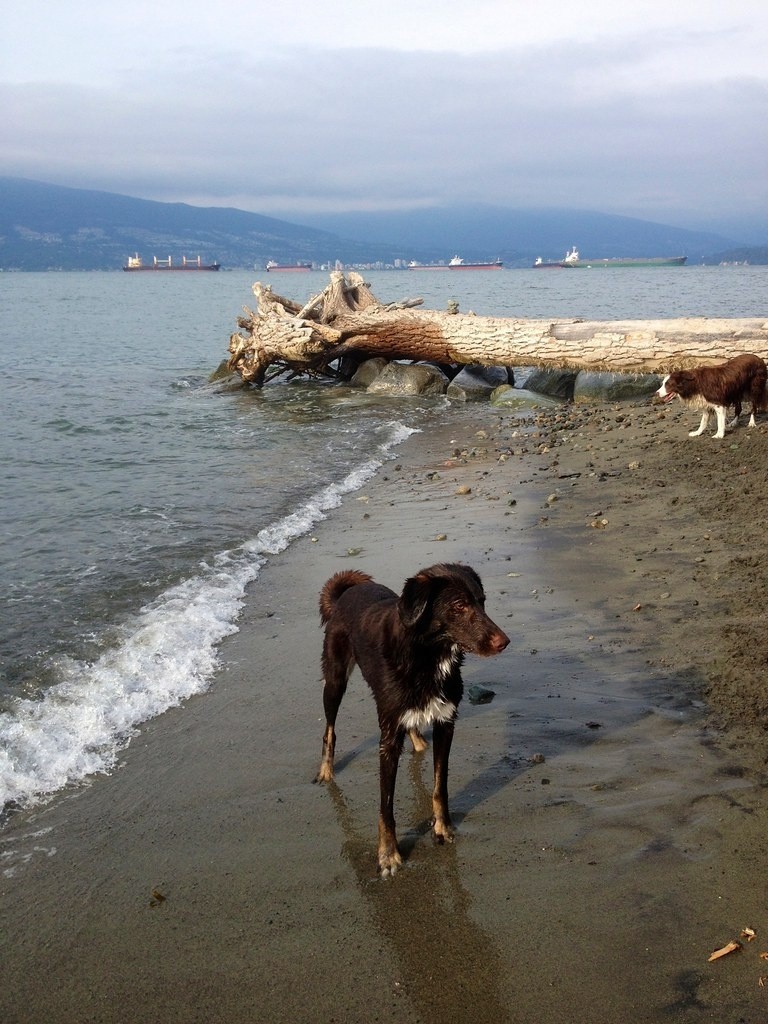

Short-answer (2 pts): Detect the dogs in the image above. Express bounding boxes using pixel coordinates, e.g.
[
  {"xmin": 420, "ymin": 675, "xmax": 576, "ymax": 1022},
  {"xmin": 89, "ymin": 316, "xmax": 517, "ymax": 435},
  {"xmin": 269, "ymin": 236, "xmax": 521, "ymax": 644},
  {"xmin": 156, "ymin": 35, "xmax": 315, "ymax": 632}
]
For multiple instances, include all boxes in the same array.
[
  {"xmin": 315, "ymin": 563, "xmax": 510, "ymax": 874},
  {"xmin": 655, "ymin": 354, "xmax": 768, "ymax": 438}
]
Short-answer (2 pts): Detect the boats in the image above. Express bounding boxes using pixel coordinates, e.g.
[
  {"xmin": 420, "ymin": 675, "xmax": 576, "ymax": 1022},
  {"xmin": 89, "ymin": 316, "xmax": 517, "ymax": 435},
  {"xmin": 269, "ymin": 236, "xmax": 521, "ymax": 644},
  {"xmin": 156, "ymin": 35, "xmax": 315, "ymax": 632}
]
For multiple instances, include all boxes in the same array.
[
  {"xmin": 123, "ymin": 257, "xmax": 221, "ymax": 271},
  {"xmin": 408, "ymin": 254, "xmax": 504, "ymax": 272},
  {"xmin": 533, "ymin": 246, "xmax": 688, "ymax": 267},
  {"xmin": 266, "ymin": 260, "xmax": 313, "ymax": 272},
  {"xmin": 719, "ymin": 260, "xmax": 750, "ymax": 266}
]
